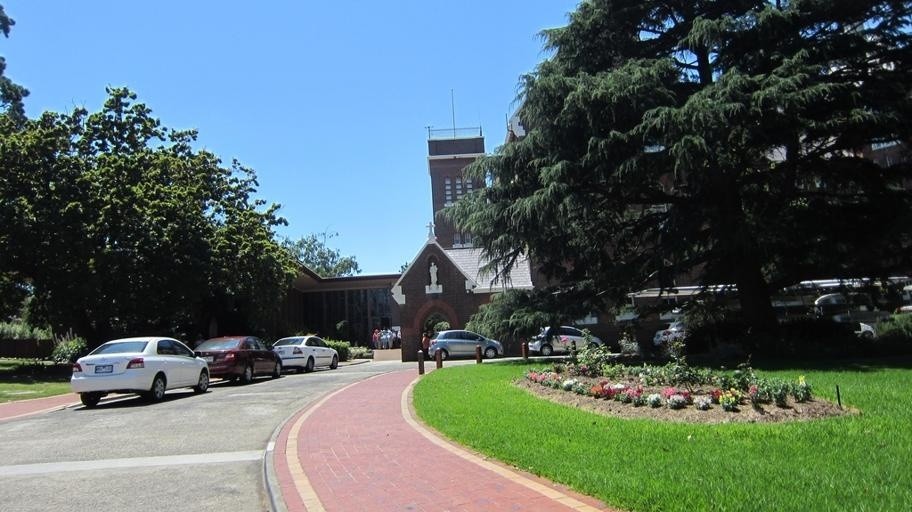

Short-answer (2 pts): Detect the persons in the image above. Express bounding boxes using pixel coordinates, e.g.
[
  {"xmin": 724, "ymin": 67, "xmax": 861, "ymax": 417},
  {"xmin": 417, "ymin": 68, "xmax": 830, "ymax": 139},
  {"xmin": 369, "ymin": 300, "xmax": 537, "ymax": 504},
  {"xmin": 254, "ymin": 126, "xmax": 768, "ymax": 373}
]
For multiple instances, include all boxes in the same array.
[
  {"xmin": 373, "ymin": 328, "xmax": 401, "ymax": 349},
  {"xmin": 422, "ymin": 333, "xmax": 429, "ymax": 360}
]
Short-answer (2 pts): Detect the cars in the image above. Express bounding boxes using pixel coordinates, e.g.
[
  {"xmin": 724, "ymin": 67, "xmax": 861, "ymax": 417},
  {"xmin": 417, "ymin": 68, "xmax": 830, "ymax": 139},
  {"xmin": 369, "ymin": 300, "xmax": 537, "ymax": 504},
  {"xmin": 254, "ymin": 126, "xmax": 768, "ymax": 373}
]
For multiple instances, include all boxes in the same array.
[
  {"xmin": 427, "ymin": 329, "xmax": 505, "ymax": 360},
  {"xmin": 527, "ymin": 325, "xmax": 603, "ymax": 356},
  {"xmin": 70, "ymin": 335, "xmax": 211, "ymax": 407},
  {"xmin": 271, "ymin": 335, "xmax": 339, "ymax": 373},
  {"xmin": 192, "ymin": 335, "xmax": 283, "ymax": 385}
]
